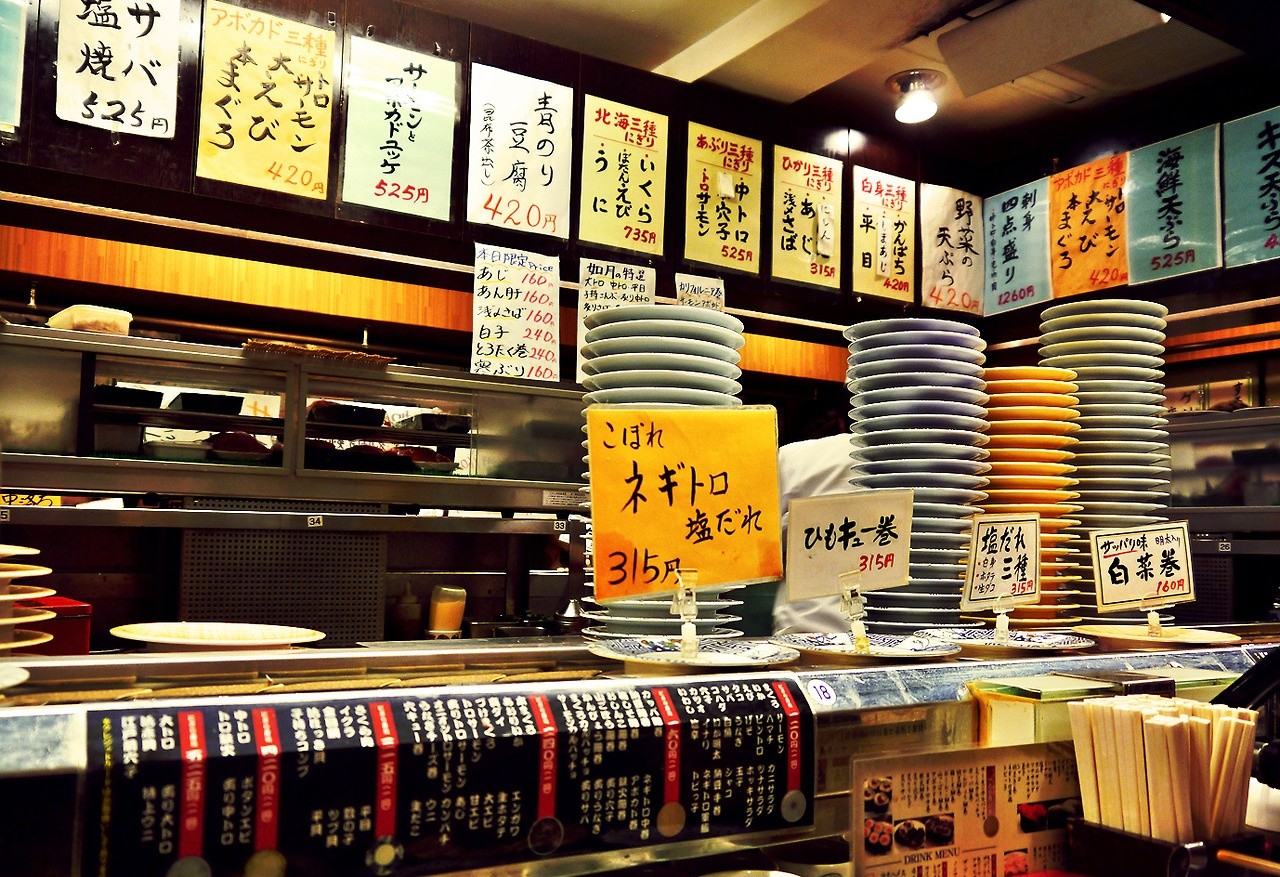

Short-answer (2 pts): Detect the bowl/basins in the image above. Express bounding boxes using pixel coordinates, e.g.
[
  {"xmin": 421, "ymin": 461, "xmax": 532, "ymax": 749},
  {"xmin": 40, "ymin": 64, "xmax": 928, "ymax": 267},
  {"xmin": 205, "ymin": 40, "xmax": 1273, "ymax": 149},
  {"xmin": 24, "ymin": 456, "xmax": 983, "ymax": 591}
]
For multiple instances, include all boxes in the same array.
[
  {"xmin": 142, "ymin": 440, "xmax": 211, "ymax": 463},
  {"xmin": 212, "ymin": 449, "xmax": 269, "ymax": 465},
  {"xmin": 93, "ymin": 384, "xmax": 163, "ymax": 409},
  {"xmin": 44, "ymin": 304, "xmax": 134, "ymax": 336},
  {"xmin": 167, "ymin": 393, "xmax": 245, "ymax": 416},
  {"xmin": 395, "ymin": 413, "xmax": 472, "ymax": 436}
]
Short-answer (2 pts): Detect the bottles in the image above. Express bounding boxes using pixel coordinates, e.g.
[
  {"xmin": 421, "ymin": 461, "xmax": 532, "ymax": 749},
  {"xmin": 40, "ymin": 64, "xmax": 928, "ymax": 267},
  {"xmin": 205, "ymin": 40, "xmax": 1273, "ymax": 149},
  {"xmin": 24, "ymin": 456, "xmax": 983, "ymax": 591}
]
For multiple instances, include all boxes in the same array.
[{"xmin": 429, "ymin": 584, "xmax": 466, "ymax": 631}]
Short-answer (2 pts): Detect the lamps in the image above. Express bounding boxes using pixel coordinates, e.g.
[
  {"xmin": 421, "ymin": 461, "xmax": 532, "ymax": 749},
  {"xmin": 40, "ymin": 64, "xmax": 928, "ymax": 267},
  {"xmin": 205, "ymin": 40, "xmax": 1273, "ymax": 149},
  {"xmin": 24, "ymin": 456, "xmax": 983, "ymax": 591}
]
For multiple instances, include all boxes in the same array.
[{"xmin": 885, "ymin": 70, "xmax": 947, "ymax": 124}]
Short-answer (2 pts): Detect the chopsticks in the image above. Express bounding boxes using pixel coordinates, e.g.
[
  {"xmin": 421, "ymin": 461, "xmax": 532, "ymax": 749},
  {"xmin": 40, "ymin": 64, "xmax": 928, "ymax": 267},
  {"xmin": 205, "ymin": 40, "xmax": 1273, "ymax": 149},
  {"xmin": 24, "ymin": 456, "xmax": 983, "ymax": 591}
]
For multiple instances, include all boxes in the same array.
[{"xmin": 1066, "ymin": 693, "xmax": 1260, "ymax": 844}]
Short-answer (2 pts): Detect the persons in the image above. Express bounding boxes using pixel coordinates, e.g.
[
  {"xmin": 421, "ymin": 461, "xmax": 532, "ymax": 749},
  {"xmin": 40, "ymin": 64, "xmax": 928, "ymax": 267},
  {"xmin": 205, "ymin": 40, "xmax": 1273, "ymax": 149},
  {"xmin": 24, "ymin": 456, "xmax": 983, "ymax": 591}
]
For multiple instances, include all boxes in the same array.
[{"xmin": 773, "ymin": 434, "xmax": 864, "ymax": 636}]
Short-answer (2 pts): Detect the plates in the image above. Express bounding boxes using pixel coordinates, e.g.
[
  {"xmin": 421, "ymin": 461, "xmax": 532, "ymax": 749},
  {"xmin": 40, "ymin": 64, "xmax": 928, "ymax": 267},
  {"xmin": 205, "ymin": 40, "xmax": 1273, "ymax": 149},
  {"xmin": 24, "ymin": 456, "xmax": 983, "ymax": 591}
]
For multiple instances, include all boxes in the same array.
[
  {"xmin": 0, "ymin": 544, "xmax": 57, "ymax": 690},
  {"xmin": 574, "ymin": 299, "xmax": 1280, "ymax": 669},
  {"xmin": 109, "ymin": 622, "xmax": 326, "ymax": 647}
]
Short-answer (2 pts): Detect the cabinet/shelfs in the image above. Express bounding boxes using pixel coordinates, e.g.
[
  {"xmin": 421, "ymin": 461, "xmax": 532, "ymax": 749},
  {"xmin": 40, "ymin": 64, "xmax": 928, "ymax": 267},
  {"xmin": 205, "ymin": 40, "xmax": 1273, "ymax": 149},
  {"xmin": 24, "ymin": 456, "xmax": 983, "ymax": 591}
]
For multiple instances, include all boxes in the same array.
[
  {"xmin": 1, "ymin": 319, "xmax": 589, "ymax": 531},
  {"xmin": 1145, "ymin": 414, "xmax": 1280, "ymax": 556}
]
